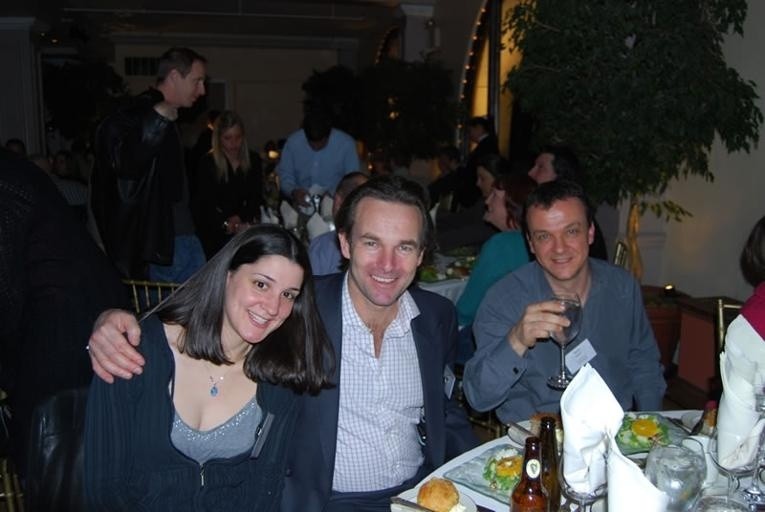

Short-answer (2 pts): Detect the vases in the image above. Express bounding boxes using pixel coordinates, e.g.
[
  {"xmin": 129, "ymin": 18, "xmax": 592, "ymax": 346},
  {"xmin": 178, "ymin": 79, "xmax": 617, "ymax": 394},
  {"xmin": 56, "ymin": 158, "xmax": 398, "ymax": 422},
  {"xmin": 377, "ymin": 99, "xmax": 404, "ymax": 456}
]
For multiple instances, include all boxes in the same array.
[{"xmin": 665, "ymin": 294, "xmax": 745, "ymax": 414}]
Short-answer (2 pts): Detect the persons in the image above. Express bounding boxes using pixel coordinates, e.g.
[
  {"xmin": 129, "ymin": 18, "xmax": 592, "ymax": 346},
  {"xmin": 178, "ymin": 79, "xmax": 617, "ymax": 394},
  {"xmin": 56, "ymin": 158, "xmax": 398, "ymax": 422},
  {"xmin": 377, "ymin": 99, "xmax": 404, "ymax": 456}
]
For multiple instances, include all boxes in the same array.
[
  {"xmin": 466, "ymin": 115, "xmax": 500, "ymax": 172},
  {"xmin": 0, "ymin": 129, "xmax": 104, "ymax": 348},
  {"xmin": 428, "ymin": 145, "xmax": 469, "ymax": 203},
  {"xmin": 79, "ymin": 221, "xmax": 335, "ymax": 512},
  {"xmin": 435, "ymin": 152, "xmax": 508, "ymax": 253},
  {"xmin": 109, "ymin": 45, "xmax": 208, "ymax": 314},
  {"xmin": 387, "ymin": 141, "xmax": 429, "ymax": 198},
  {"xmin": 204, "ymin": 109, "xmax": 265, "ymax": 260},
  {"xmin": 307, "ymin": 172, "xmax": 371, "ymax": 276},
  {"xmin": 278, "ymin": 96, "xmax": 360, "ymax": 215},
  {"xmin": 463, "ymin": 179, "xmax": 666, "ymax": 429},
  {"xmin": 724, "ymin": 214, "xmax": 765, "ymax": 396},
  {"xmin": 527, "ymin": 146, "xmax": 608, "ymax": 264},
  {"xmin": 87, "ymin": 174, "xmax": 479, "ymax": 512},
  {"xmin": 454, "ymin": 172, "xmax": 531, "ymax": 326}
]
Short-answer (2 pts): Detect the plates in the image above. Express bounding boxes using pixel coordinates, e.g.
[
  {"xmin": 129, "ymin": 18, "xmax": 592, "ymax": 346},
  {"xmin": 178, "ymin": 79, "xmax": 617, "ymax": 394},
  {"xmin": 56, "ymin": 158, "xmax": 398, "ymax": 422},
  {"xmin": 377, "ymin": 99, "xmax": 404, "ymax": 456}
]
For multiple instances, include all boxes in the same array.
[
  {"xmin": 507, "ymin": 420, "xmax": 566, "ymax": 448},
  {"xmin": 615, "ymin": 412, "xmax": 690, "ymax": 455},
  {"xmin": 402, "ymin": 491, "xmax": 478, "ymax": 512},
  {"xmin": 682, "ymin": 409, "xmax": 702, "ymax": 428},
  {"xmin": 442, "ymin": 444, "xmax": 524, "ymax": 506}
]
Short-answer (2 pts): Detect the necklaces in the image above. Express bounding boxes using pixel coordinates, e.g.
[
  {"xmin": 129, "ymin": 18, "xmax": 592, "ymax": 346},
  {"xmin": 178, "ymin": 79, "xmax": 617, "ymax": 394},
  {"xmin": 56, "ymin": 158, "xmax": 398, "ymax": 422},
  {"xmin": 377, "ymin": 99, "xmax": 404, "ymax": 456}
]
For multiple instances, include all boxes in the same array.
[{"xmin": 205, "ymin": 359, "xmax": 231, "ymax": 396}]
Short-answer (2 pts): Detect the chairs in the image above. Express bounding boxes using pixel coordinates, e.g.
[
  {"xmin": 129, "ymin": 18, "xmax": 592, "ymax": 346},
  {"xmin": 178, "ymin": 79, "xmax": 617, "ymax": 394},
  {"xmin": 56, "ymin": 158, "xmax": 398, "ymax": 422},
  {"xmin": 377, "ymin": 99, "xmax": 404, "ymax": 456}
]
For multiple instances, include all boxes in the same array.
[
  {"xmin": 125, "ymin": 280, "xmax": 185, "ymax": 318},
  {"xmin": 715, "ymin": 300, "xmax": 753, "ymax": 404}
]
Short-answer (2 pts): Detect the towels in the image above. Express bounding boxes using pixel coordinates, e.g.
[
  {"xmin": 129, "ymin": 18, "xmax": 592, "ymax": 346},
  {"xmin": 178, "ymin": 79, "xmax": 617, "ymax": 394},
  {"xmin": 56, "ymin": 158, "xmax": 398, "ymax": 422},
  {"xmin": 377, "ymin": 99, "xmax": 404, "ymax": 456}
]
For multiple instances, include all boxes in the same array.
[
  {"xmin": 321, "ymin": 195, "xmax": 333, "ymax": 215},
  {"xmin": 602, "ymin": 426, "xmax": 668, "ymax": 512},
  {"xmin": 717, "ymin": 347, "xmax": 765, "ymax": 469},
  {"xmin": 306, "ymin": 213, "xmax": 329, "ymax": 239},
  {"xmin": 280, "ymin": 200, "xmax": 298, "ymax": 228},
  {"xmin": 559, "ymin": 361, "xmax": 625, "ymax": 496}
]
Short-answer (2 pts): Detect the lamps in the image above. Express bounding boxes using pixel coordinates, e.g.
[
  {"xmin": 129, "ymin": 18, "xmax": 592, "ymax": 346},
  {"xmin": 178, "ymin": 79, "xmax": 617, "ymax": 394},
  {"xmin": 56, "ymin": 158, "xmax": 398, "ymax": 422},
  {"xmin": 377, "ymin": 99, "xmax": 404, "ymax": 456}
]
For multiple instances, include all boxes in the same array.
[
  {"xmin": 454, "ymin": 2, "xmax": 487, "ymax": 166},
  {"xmin": 41, "ymin": 22, "xmax": 78, "ymax": 50},
  {"xmin": 247, "ymin": 111, "xmax": 289, "ymax": 164},
  {"xmin": 664, "ymin": 282, "xmax": 676, "ymax": 295}
]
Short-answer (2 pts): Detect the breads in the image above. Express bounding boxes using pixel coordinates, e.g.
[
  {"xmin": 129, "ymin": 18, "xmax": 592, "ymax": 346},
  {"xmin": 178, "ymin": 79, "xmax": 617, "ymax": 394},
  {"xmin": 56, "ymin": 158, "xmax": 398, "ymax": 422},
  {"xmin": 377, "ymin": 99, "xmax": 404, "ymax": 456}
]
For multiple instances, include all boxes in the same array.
[{"xmin": 416, "ymin": 476, "xmax": 460, "ymax": 511}]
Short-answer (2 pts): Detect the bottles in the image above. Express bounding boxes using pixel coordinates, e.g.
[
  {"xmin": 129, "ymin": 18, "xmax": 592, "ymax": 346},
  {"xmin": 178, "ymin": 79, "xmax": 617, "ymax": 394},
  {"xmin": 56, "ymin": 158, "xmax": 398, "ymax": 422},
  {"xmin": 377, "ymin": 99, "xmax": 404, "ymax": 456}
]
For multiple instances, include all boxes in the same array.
[
  {"xmin": 538, "ymin": 415, "xmax": 562, "ymax": 512},
  {"xmin": 509, "ymin": 435, "xmax": 552, "ymax": 511},
  {"xmin": 292, "ymin": 227, "xmax": 300, "ymax": 240}
]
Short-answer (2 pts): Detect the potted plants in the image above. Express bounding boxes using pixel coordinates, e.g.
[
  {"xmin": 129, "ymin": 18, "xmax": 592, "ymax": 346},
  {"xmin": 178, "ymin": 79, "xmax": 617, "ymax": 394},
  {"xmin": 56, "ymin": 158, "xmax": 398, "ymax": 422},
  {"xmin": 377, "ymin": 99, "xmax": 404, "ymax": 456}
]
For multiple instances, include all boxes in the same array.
[{"xmin": 492, "ymin": 0, "xmax": 687, "ymax": 380}]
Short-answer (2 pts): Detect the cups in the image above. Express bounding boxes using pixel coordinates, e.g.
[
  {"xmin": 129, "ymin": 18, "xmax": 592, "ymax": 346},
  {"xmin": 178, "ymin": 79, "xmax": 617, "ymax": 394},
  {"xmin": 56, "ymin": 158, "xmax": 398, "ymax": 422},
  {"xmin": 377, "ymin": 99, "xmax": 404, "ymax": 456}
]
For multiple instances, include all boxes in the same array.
[
  {"xmin": 546, "ymin": 293, "xmax": 583, "ymax": 388},
  {"xmin": 646, "ymin": 436, "xmax": 705, "ymax": 511}
]
